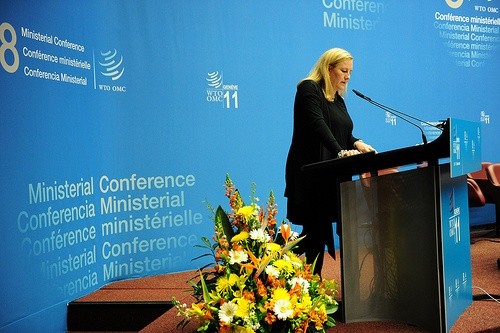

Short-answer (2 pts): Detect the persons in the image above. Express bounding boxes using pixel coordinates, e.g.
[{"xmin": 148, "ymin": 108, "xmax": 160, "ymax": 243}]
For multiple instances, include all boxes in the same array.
[{"xmin": 284, "ymin": 48, "xmax": 378, "ymax": 279}]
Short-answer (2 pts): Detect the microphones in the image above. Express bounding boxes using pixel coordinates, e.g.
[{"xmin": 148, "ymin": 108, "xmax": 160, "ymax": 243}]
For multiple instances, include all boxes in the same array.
[{"xmin": 352, "ymin": 89, "xmax": 442, "ymax": 144}]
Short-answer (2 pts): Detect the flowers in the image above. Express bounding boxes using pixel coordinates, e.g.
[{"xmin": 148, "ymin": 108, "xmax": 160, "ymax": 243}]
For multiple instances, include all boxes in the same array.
[{"xmin": 171, "ymin": 173, "xmax": 339, "ymax": 333}]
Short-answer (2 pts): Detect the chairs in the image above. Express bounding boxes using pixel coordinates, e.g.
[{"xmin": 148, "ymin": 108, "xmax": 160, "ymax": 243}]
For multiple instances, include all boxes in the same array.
[
  {"xmin": 486, "ymin": 163, "xmax": 500, "ymax": 269},
  {"xmin": 466, "ymin": 179, "xmax": 486, "ymax": 208}
]
